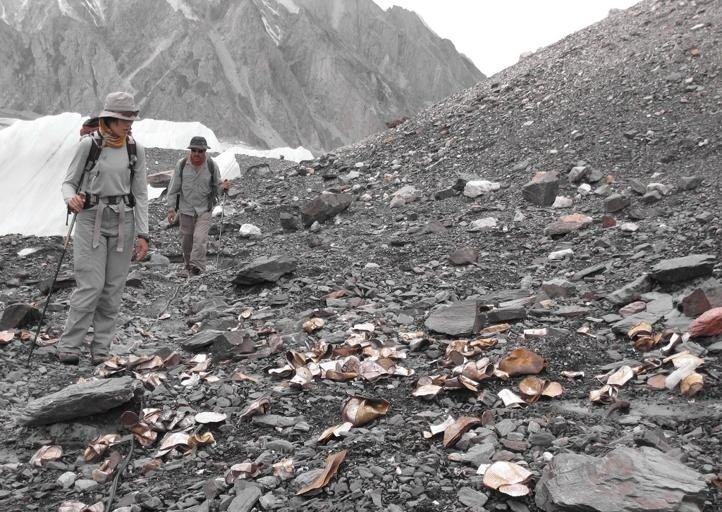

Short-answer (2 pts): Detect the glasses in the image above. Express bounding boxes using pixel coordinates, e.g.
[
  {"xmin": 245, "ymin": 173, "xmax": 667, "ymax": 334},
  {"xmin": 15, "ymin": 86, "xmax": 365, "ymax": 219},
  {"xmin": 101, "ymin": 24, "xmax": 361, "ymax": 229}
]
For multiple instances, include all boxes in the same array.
[
  {"xmin": 103, "ymin": 108, "xmax": 140, "ymax": 116},
  {"xmin": 191, "ymin": 150, "xmax": 203, "ymax": 153}
]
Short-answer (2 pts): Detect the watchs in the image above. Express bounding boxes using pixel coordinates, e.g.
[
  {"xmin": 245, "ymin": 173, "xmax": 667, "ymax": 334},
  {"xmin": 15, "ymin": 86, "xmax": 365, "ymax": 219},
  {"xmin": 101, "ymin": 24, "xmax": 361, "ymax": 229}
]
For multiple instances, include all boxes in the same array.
[{"xmin": 137, "ymin": 234, "xmax": 151, "ymax": 242}]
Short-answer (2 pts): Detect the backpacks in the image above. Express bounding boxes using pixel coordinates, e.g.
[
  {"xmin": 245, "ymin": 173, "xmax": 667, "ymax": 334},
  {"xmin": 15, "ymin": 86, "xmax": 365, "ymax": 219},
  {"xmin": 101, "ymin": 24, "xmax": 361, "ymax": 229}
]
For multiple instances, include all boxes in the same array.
[{"xmin": 80, "ymin": 117, "xmax": 136, "ymax": 176}]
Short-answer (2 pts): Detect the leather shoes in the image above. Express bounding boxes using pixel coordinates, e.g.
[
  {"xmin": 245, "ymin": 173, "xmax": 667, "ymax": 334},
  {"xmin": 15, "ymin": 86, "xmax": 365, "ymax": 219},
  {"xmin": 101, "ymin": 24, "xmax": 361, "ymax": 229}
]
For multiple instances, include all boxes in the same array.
[
  {"xmin": 56, "ymin": 351, "xmax": 80, "ymax": 365},
  {"xmin": 91, "ymin": 352, "xmax": 110, "ymax": 366}
]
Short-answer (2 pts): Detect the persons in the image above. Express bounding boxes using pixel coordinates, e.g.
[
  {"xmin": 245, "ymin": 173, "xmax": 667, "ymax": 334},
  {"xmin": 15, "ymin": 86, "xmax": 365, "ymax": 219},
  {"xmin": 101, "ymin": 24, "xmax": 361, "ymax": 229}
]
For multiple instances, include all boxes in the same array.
[
  {"xmin": 56, "ymin": 92, "xmax": 149, "ymax": 366},
  {"xmin": 167, "ymin": 137, "xmax": 231, "ymax": 276}
]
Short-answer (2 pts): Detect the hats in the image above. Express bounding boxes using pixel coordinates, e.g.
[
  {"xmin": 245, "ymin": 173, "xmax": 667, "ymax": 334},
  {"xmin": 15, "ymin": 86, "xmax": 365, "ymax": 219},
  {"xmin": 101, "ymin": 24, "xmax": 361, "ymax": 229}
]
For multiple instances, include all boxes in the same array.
[
  {"xmin": 97, "ymin": 91, "xmax": 141, "ymax": 121},
  {"xmin": 186, "ymin": 136, "xmax": 211, "ymax": 150}
]
